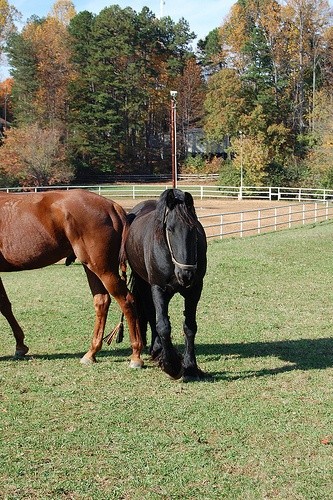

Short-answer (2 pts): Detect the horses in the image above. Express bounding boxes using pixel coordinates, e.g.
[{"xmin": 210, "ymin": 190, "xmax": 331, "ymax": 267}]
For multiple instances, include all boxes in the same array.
[
  {"xmin": 102, "ymin": 188, "xmax": 213, "ymax": 383},
  {"xmin": 0, "ymin": 190, "xmax": 144, "ymax": 371}
]
